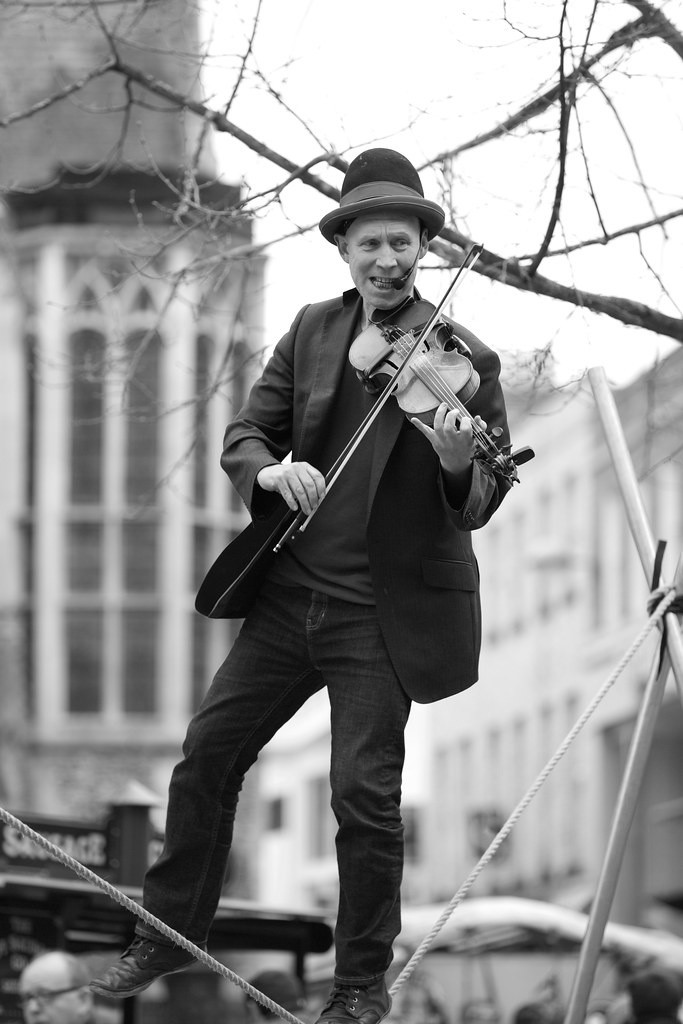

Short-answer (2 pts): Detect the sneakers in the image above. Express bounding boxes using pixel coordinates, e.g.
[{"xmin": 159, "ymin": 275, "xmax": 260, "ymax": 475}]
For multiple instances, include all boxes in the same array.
[
  {"xmin": 89, "ymin": 935, "xmax": 208, "ymax": 1000},
  {"xmin": 315, "ymin": 976, "xmax": 393, "ymax": 1024}
]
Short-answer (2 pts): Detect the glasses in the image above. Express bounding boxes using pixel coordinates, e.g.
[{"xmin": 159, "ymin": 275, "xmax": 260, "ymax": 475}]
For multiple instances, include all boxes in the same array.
[{"xmin": 16, "ymin": 984, "xmax": 83, "ymax": 1009}]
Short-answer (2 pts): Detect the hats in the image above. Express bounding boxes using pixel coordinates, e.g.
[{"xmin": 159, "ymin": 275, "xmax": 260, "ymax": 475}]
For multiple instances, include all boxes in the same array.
[{"xmin": 319, "ymin": 148, "xmax": 445, "ymax": 245}]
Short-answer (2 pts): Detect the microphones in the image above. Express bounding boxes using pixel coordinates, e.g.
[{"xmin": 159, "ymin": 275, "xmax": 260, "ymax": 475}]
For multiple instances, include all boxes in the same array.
[{"xmin": 394, "ymin": 239, "xmax": 423, "ymax": 285}]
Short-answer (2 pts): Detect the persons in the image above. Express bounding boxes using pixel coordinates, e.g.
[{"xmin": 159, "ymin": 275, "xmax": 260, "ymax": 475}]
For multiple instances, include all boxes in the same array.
[
  {"xmin": 92, "ymin": 147, "xmax": 514, "ymax": 1024},
  {"xmin": 0, "ymin": 950, "xmax": 683, "ymax": 1024}
]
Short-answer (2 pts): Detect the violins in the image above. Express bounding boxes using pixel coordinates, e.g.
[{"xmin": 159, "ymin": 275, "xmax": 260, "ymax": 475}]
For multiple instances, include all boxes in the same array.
[{"xmin": 347, "ymin": 299, "xmax": 535, "ymax": 487}]
